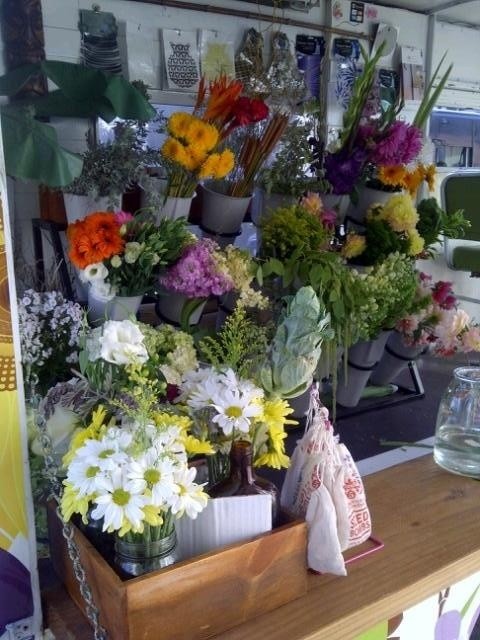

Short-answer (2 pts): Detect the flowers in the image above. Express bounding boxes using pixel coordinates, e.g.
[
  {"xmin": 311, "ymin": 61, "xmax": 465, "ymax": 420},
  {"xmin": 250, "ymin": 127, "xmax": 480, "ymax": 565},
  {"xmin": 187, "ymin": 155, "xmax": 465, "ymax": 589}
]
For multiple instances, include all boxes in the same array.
[{"xmin": 24, "ymin": 37, "xmax": 478, "ymax": 573}]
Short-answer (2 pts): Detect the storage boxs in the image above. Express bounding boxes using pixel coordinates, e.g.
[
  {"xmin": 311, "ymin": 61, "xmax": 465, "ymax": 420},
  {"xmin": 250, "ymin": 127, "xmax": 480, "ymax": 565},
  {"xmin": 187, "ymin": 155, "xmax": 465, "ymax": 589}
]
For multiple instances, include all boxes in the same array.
[{"xmin": 48, "ymin": 503, "xmax": 312, "ymax": 637}]
